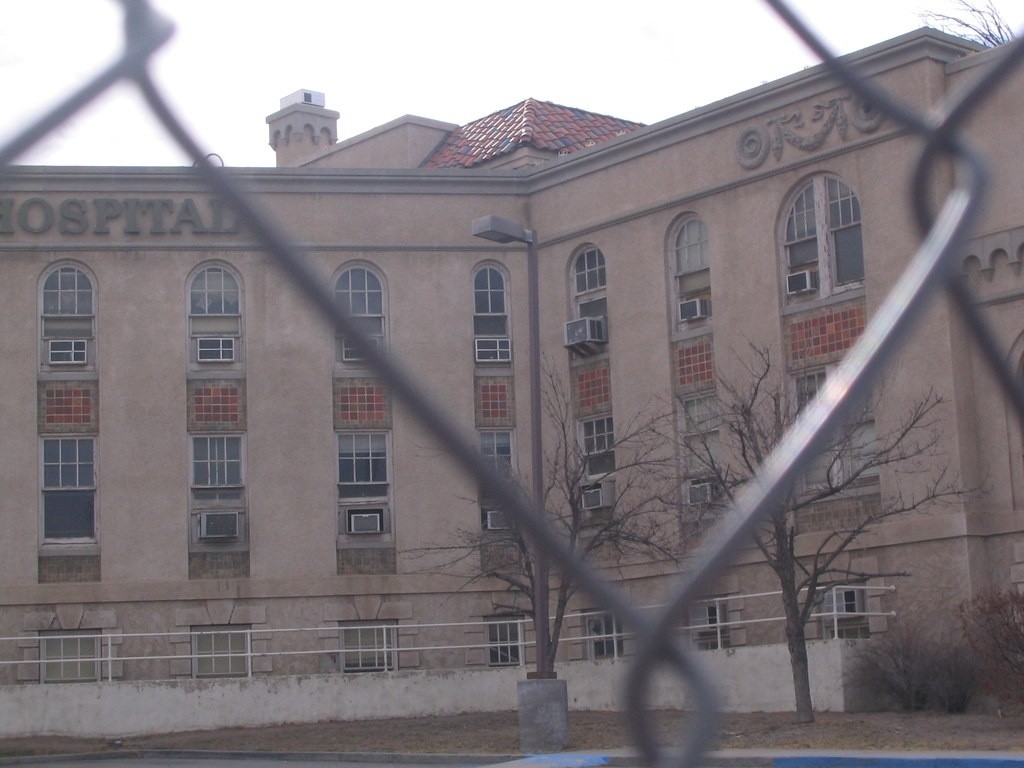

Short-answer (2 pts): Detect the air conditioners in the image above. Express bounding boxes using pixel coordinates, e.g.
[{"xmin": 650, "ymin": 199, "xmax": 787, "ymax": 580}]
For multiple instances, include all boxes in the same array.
[
  {"xmin": 200, "ymin": 512, "xmax": 239, "ymax": 537},
  {"xmin": 486, "ymin": 511, "xmax": 516, "ymax": 530},
  {"xmin": 563, "ymin": 318, "xmax": 607, "ymax": 346},
  {"xmin": 582, "ymin": 489, "xmax": 605, "ymax": 508},
  {"xmin": 351, "ymin": 514, "xmax": 381, "ymax": 534},
  {"xmin": 679, "ymin": 297, "xmax": 710, "ymax": 321},
  {"xmin": 787, "ymin": 271, "xmax": 818, "ymax": 294},
  {"xmin": 688, "ymin": 483, "xmax": 719, "ymax": 505}
]
